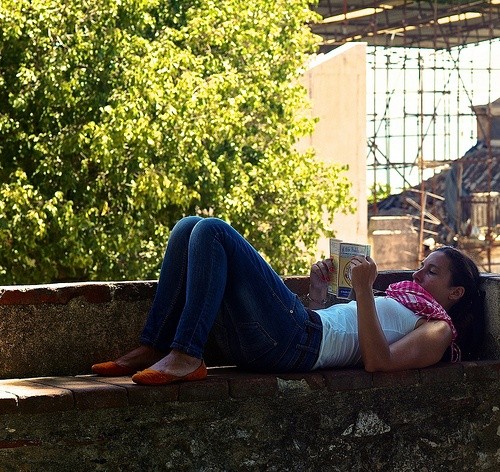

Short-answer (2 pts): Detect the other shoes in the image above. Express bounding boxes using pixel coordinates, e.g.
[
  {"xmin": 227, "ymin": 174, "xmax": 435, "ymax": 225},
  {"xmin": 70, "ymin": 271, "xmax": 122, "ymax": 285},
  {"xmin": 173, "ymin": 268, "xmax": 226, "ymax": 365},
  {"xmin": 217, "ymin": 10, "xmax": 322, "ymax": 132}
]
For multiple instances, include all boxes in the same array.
[
  {"xmin": 132, "ymin": 362, "xmax": 208, "ymax": 384},
  {"xmin": 91, "ymin": 359, "xmax": 155, "ymax": 375}
]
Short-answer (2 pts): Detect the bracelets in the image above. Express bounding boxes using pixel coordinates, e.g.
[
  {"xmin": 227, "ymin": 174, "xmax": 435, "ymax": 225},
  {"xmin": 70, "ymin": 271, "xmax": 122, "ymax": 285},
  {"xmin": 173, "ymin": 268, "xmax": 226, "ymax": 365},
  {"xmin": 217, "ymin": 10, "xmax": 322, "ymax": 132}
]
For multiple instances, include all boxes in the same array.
[{"xmin": 306, "ymin": 292, "xmax": 326, "ymax": 308}]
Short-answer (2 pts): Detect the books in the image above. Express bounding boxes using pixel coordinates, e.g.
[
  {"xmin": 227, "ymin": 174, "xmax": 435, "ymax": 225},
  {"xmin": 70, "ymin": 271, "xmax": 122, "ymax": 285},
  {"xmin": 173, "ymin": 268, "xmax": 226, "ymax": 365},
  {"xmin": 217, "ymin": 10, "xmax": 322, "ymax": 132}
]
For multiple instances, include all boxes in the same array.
[{"xmin": 327, "ymin": 238, "xmax": 371, "ymax": 303}]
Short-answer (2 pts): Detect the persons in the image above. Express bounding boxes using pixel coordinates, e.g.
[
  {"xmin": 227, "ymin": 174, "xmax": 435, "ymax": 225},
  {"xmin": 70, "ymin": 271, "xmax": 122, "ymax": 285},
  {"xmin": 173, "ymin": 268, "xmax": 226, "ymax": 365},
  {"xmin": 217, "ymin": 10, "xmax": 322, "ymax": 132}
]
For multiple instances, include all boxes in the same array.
[{"xmin": 91, "ymin": 216, "xmax": 482, "ymax": 387}]
